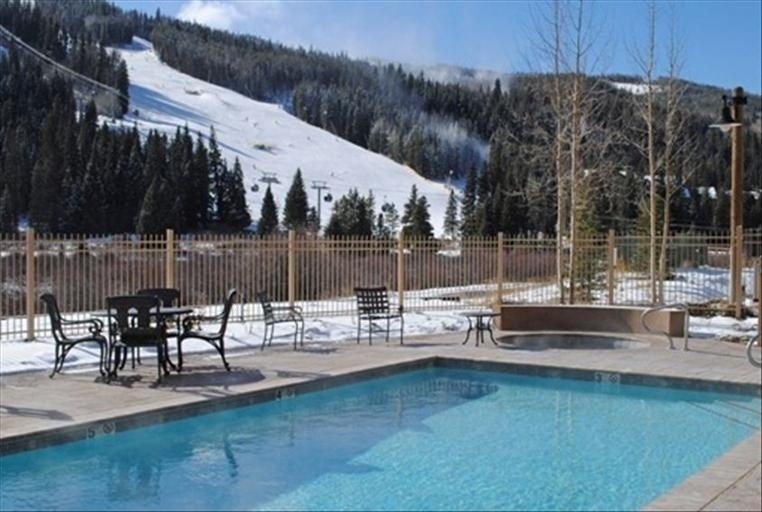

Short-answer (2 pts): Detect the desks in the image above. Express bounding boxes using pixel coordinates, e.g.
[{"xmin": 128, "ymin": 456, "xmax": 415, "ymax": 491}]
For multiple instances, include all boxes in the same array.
[{"xmin": 453, "ymin": 311, "xmax": 502, "ymax": 347}]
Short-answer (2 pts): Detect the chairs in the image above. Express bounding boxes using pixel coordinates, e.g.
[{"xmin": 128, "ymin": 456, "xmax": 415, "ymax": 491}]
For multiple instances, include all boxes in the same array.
[
  {"xmin": 38, "ymin": 287, "xmax": 236, "ymax": 384},
  {"xmin": 256, "ymin": 289, "xmax": 304, "ymax": 352},
  {"xmin": 353, "ymin": 286, "xmax": 404, "ymax": 346}
]
